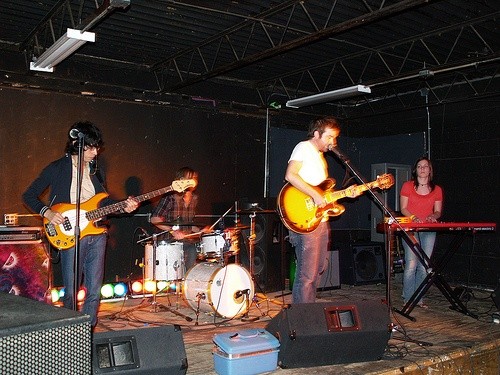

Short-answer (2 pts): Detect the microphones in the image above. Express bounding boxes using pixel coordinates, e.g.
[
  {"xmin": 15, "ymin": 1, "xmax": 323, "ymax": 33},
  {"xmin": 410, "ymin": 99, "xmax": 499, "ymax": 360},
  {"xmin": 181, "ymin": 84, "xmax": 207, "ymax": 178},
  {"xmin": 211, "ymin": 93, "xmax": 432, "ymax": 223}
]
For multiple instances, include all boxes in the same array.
[
  {"xmin": 69, "ymin": 128, "xmax": 83, "ymax": 139},
  {"xmin": 327, "ymin": 144, "xmax": 344, "ymax": 159}
]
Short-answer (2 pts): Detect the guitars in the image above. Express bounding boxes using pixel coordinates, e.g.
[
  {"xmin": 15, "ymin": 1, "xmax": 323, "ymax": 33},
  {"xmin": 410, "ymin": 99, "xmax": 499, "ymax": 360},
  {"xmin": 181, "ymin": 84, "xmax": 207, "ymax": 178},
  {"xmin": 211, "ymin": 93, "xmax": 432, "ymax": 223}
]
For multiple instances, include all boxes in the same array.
[
  {"xmin": 277, "ymin": 172, "xmax": 394, "ymax": 234},
  {"xmin": 42, "ymin": 178, "xmax": 196, "ymax": 250}
]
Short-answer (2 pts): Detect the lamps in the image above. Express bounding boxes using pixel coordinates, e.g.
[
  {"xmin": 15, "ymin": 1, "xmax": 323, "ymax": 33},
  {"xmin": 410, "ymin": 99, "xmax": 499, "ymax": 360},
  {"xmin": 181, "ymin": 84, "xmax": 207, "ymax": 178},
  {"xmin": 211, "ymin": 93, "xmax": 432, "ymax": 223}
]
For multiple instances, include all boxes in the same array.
[
  {"xmin": 285, "ymin": 85, "xmax": 371, "ymax": 108},
  {"xmin": 50, "ymin": 279, "xmax": 177, "ymax": 306},
  {"xmin": 29, "ymin": 28, "xmax": 95, "ymax": 73}
]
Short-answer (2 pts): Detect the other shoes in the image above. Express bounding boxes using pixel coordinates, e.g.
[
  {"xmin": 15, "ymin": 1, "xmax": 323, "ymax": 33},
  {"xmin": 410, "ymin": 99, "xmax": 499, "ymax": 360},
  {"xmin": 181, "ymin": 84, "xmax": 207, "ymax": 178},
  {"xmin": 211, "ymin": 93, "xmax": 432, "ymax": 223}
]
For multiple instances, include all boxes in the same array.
[
  {"xmin": 400, "ymin": 301, "xmax": 411, "ymax": 313},
  {"xmin": 415, "ymin": 303, "xmax": 428, "ymax": 310}
]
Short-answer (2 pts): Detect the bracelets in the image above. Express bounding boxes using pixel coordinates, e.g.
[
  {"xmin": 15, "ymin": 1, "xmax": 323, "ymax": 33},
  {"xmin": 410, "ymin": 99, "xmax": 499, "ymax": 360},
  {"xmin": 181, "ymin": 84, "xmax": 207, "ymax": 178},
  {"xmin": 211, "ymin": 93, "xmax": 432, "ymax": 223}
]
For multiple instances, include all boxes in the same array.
[
  {"xmin": 170, "ymin": 231, "xmax": 174, "ymax": 233},
  {"xmin": 411, "ymin": 215, "xmax": 415, "ymax": 219}
]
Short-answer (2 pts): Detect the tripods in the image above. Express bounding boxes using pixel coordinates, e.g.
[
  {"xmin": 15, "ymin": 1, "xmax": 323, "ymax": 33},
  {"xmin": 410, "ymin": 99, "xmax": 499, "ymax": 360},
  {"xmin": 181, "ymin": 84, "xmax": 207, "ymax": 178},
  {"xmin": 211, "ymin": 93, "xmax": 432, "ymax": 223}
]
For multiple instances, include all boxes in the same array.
[
  {"xmin": 110, "ymin": 226, "xmax": 193, "ymax": 324},
  {"xmin": 237, "ymin": 211, "xmax": 280, "ymax": 318}
]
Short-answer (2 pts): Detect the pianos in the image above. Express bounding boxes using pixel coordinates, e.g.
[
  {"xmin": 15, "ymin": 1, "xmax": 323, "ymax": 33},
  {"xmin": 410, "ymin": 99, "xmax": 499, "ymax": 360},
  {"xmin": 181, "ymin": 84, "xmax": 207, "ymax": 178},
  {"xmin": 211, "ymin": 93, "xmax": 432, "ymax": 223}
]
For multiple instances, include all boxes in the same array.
[{"xmin": 376, "ymin": 217, "xmax": 498, "ymax": 324}]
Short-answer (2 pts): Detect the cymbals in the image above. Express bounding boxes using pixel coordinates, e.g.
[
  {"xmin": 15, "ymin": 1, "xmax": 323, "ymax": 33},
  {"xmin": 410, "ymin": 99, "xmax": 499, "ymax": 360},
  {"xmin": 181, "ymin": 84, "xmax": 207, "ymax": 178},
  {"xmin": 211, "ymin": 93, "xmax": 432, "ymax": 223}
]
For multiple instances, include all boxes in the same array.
[
  {"xmin": 153, "ymin": 219, "xmax": 207, "ymax": 226},
  {"xmin": 234, "ymin": 209, "xmax": 276, "ymax": 213},
  {"xmin": 227, "ymin": 226, "xmax": 250, "ymax": 229}
]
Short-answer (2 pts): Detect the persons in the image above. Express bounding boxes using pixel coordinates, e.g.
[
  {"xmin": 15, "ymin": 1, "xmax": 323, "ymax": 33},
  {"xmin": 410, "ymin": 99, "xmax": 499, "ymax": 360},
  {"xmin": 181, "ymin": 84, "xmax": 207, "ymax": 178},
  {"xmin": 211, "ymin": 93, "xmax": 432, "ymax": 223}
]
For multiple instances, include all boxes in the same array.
[
  {"xmin": 284, "ymin": 117, "xmax": 358, "ymax": 305},
  {"xmin": 20, "ymin": 122, "xmax": 138, "ymax": 334},
  {"xmin": 400, "ymin": 158, "xmax": 442, "ymax": 312},
  {"xmin": 150, "ymin": 167, "xmax": 203, "ymax": 272}
]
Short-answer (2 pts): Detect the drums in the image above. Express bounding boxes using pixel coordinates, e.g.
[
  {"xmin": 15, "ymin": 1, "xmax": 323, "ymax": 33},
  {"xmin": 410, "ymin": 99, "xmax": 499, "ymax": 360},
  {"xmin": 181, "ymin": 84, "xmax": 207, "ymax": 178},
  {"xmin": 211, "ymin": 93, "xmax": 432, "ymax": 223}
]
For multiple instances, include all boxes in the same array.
[
  {"xmin": 200, "ymin": 229, "xmax": 241, "ymax": 262},
  {"xmin": 183, "ymin": 261, "xmax": 254, "ymax": 319},
  {"xmin": 143, "ymin": 241, "xmax": 186, "ymax": 283}
]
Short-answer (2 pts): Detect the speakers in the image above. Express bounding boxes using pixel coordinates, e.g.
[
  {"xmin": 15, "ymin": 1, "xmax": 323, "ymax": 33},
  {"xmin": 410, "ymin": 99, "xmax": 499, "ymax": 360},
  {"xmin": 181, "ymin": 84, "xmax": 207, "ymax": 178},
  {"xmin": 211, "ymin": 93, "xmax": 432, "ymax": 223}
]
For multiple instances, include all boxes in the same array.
[
  {"xmin": 238, "ymin": 214, "xmax": 283, "ymax": 294},
  {"xmin": 0, "ymin": 291, "xmax": 92, "ymax": 375},
  {"xmin": 89, "ymin": 324, "xmax": 188, "ymax": 375},
  {"xmin": 329, "ymin": 241, "xmax": 387, "ymax": 286},
  {"xmin": 266, "ymin": 298, "xmax": 393, "ymax": 370}
]
